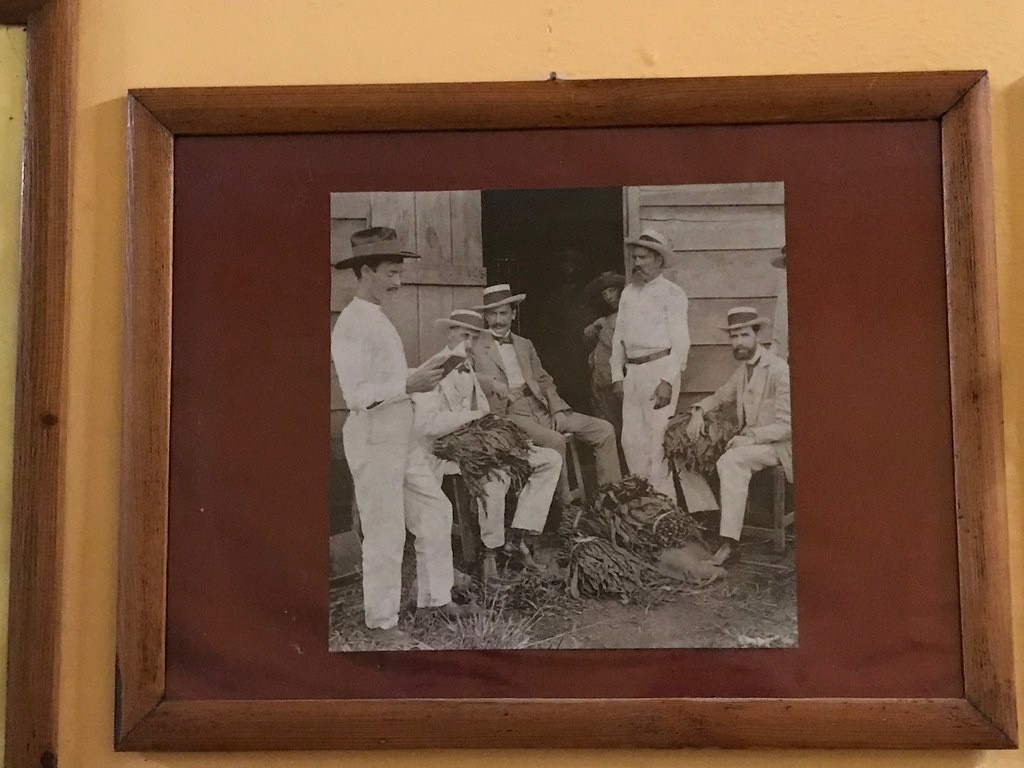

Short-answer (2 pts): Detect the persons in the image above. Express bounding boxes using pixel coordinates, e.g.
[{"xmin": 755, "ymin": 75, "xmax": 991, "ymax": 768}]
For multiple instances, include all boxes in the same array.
[
  {"xmin": 329, "ymin": 226, "xmax": 468, "ymax": 646},
  {"xmin": 608, "ymin": 229, "xmax": 691, "ymax": 505},
  {"xmin": 408, "ymin": 307, "xmax": 563, "ymax": 591},
  {"xmin": 468, "ymin": 282, "xmax": 623, "ymax": 537},
  {"xmin": 578, "ymin": 270, "xmax": 626, "ymax": 479},
  {"xmin": 685, "ymin": 306, "xmax": 790, "ymax": 567}
]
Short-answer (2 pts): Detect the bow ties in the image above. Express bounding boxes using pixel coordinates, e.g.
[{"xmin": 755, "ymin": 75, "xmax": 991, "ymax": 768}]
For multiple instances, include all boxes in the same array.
[
  {"xmin": 491, "ymin": 332, "xmax": 513, "ymax": 346},
  {"xmin": 458, "ymin": 365, "xmax": 470, "ymax": 373}
]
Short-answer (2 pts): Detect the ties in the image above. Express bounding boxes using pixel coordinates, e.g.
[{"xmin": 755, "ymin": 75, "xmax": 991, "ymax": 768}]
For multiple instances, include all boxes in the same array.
[{"xmin": 745, "ymin": 358, "xmax": 760, "ymax": 384}]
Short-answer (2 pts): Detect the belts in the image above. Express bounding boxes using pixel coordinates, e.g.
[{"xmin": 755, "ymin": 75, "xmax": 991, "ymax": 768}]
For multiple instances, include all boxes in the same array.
[
  {"xmin": 627, "ymin": 348, "xmax": 671, "ymax": 365},
  {"xmin": 507, "ymin": 388, "xmax": 534, "ymax": 406}
]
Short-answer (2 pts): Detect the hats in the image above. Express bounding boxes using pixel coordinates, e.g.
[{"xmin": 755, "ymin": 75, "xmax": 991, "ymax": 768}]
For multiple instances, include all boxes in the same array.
[
  {"xmin": 470, "ymin": 284, "xmax": 526, "ymax": 311},
  {"xmin": 434, "ymin": 309, "xmax": 494, "ymax": 334},
  {"xmin": 624, "ymin": 230, "xmax": 674, "ymax": 269},
  {"xmin": 717, "ymin": 307, "xmax": 771, "ymax": 333},
  {"xmin": 334, "ymin": 227, "xmax": 422, "ymax": 270},
  {"xmin": 587, "ymin": 272, "xmax": 625, "ymax": 293}
]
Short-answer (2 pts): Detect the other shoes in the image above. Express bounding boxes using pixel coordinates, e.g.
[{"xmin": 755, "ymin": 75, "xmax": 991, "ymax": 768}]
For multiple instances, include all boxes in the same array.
[
  {"xmin": 366, "ymin": 626, "xmax": 405, "ymax": 640},
  {"xmin": 479, "ymin": 545, "xmax": 499, "ymax": 587},
  {"xmin": 415, "ymin": 602, "xmax": 470, "ymax": 621},
  {"xmin": 707, "ymin": 540, "xmax": 742, "ymax": 567},
  {"xmin": 500, "ymin": 539, "xmax": 537, "ymax": 570}
]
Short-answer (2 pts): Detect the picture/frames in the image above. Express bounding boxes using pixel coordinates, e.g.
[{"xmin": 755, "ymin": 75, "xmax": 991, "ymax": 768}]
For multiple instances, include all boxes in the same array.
[{"xmin": 112, "ymin": 70, "xmax": 1018, "ymax": 750}]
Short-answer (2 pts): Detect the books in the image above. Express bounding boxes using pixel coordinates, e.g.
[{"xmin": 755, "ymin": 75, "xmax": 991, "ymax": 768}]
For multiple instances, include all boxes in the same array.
[{"xmin": 420, "ymin": 341, "xmax": 467, "ymax": 381}]
